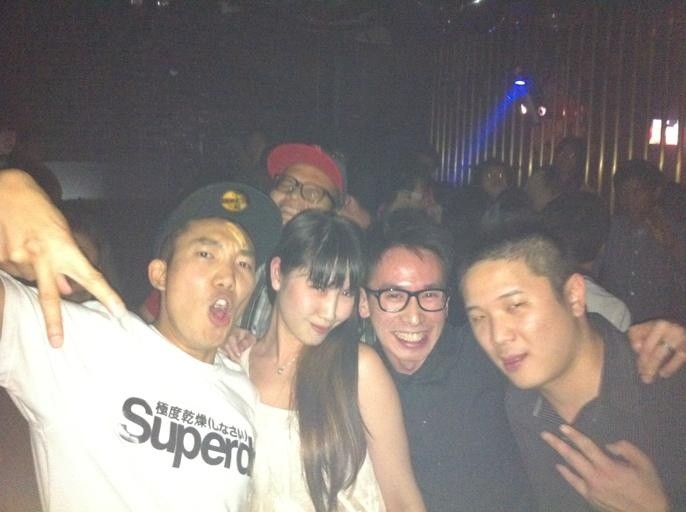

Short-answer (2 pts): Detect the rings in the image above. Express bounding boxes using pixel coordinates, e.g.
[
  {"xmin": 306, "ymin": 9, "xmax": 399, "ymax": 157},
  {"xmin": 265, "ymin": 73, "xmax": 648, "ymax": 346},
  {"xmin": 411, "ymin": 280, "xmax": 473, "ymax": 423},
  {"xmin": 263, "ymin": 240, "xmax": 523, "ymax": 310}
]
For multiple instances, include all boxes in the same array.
[{"xmin": 658, "ymin": 339, "xmax": 675, "ymax": 356}]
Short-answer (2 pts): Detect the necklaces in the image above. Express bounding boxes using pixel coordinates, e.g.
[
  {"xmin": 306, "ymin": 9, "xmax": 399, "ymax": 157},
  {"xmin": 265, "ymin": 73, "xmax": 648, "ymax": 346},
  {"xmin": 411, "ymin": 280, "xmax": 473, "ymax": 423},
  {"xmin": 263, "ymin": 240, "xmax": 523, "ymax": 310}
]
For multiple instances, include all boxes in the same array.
[{"xmin": 267, "ymin": 333, "xmax": 301, "ymax": 375}]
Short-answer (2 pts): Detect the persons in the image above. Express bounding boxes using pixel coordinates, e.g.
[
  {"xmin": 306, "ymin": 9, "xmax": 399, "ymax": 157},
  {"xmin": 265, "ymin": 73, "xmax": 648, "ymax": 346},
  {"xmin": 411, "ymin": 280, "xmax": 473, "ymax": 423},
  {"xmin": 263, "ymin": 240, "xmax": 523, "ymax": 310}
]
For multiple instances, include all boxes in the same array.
[
  {"xmin": 454, "ymin": 228, "xmax": 686, "ymax": 512},
  {"xmin": 140, "ymin": 143, "xmax": 345, "ymax": 342},
  {"xmin": 0, "ymin": 168, "xmax": 279, "ymax": 512},
  {"xmin": 356, "ymin": 229, "xmax": 479, "ymax": 512},
  {"xmin": 345, "ymin": 140, "xmax": 686, "ymax": 332},
  {"xmin": 234, "ymin": 209, "xmax": 422, "ymax": 511}
]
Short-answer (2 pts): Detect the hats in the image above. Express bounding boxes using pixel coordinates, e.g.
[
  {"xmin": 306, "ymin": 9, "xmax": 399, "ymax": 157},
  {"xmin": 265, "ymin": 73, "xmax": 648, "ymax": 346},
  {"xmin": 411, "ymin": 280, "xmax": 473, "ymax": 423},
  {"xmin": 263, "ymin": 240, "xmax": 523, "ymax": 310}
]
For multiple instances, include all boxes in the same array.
[
  {"xmin": 152, "ymin": 182, "xmax": 282, "ymax": 272},
  {"xmin": 266, "ymin": 144, "xmax": 344, "ymax": 201}
]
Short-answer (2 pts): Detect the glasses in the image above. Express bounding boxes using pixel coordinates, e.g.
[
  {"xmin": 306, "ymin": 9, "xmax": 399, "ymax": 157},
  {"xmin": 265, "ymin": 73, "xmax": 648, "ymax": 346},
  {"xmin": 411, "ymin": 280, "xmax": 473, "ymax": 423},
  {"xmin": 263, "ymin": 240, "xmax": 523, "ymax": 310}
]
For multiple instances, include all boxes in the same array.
[
  {"xmin": 276, "ymin": 175, "xmax": 335, "ymax": 205},
  {"xmin": 365, "ymin": 288, "xmax": 451, "ymax": 313}
]
